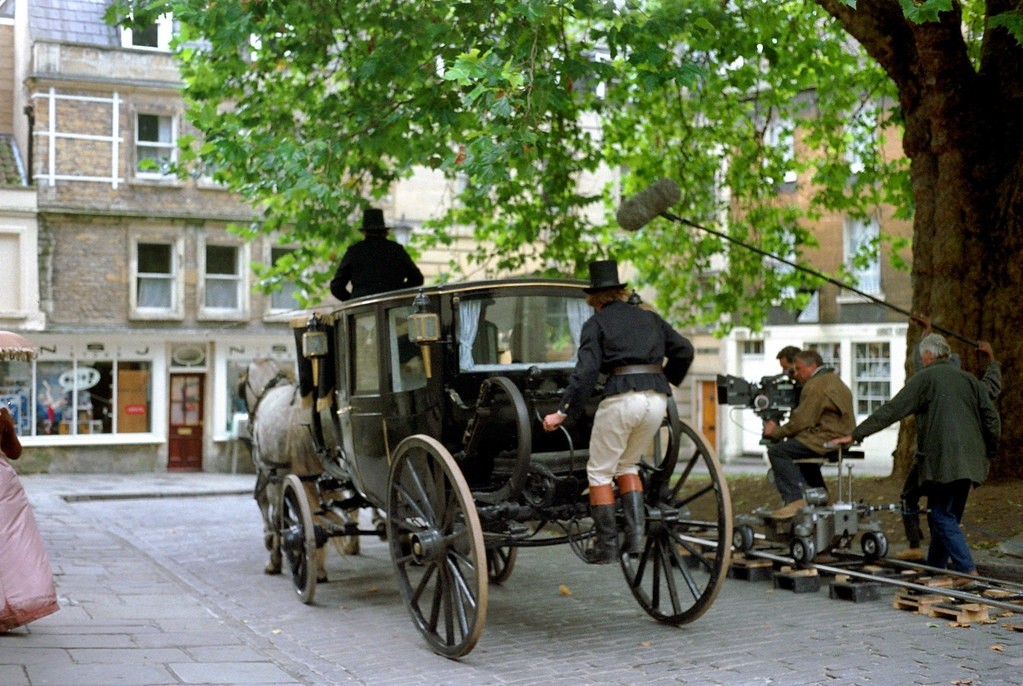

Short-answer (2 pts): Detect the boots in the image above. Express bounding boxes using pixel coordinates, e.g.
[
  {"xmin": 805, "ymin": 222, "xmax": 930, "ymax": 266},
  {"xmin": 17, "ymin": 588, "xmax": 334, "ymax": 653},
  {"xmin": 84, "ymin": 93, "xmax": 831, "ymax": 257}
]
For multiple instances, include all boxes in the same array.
[
  {"xmin": 618, "ymin": 473, "xmax": 648, "ymax": 557},
  {"xmin": 586, "ymin": 485, "xmax": 616, "ymax": 563}
]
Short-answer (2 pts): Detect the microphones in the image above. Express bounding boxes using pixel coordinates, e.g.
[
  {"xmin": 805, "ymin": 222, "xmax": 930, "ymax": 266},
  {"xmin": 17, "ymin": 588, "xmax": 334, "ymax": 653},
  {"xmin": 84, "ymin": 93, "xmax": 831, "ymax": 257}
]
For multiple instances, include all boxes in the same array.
[{"xmin": 616, "ymin": 178, "xmax": 681, "ymax": 233}]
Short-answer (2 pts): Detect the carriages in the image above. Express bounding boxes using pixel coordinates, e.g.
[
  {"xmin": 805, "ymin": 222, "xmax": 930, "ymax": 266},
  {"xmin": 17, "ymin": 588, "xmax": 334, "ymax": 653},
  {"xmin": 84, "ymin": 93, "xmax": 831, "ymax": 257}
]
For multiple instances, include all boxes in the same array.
[{"xmin": 236, "ymin": 275, "xmax": 734, "ymax": 660}]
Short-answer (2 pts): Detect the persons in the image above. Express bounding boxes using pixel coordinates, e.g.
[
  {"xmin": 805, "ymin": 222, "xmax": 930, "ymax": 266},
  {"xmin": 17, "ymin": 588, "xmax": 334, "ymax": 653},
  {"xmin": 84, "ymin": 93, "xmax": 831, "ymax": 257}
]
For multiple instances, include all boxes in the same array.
[
  {"xmin": 762, "ymin": 346, "xmax": 857, "ymax": 519},
  {"xmin": 0, "ymin": 407, "xmax": 60, "ymax": 634},
  {"xmin": 830, "ymin": 316, "xmax": 1003, "ymax": 588},
  {"xmin": 329, "ymin": 207, "xmax": 425, "ymax": 302},
  {"xmin": 542, "ymin": 260, "xmax": 694, "ymax": 566}
]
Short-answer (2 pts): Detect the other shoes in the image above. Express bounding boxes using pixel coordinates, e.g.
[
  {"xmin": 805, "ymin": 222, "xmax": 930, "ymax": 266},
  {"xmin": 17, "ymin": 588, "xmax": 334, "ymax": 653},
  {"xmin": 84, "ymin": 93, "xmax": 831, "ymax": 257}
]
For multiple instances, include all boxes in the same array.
[
  {"xmin": 954, "ymin": 572, "xmax": 978, "ymax": 586},
  {"xmin": 770, "ymin": 499, "xmax": 806, "ymax": 519},
  {"xmin": 897, "ymin": 548, "xmax": 924, "ymax": 559}
]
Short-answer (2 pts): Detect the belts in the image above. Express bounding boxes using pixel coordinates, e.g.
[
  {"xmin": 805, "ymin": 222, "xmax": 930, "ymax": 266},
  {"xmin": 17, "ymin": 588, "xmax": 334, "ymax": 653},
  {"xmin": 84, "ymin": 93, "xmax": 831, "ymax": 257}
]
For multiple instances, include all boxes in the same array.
[{"xmin": 609, "ymin": 364, "xmax": 665, "ymax": 376}]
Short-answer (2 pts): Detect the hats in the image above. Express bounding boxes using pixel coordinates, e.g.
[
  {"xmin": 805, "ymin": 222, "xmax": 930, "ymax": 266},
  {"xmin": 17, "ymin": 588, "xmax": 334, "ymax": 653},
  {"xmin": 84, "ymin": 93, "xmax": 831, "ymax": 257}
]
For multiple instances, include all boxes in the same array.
[
  {"xmin": 583, "ymin": 261, "xmax": 628, "ymax": 294},
  {"xmin": 356, "ymin": 209, "xmax": 391, "ymax": 231}
]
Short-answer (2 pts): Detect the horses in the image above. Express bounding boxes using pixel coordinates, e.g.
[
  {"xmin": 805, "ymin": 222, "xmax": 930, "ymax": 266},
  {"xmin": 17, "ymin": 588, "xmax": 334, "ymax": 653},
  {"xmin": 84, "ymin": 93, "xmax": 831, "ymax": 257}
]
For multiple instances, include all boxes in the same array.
[{"xmin": 238, "ymin": 357, "xmax": 387, "ymax": 584}]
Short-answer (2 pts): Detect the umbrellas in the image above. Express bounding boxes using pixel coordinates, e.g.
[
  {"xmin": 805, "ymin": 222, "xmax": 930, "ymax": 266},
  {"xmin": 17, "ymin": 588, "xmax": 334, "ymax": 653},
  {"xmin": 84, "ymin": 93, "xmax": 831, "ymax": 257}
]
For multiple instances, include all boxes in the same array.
[{"xmin": 0, "ymin": 330, "xmax": 38, "ymax": 362}]
